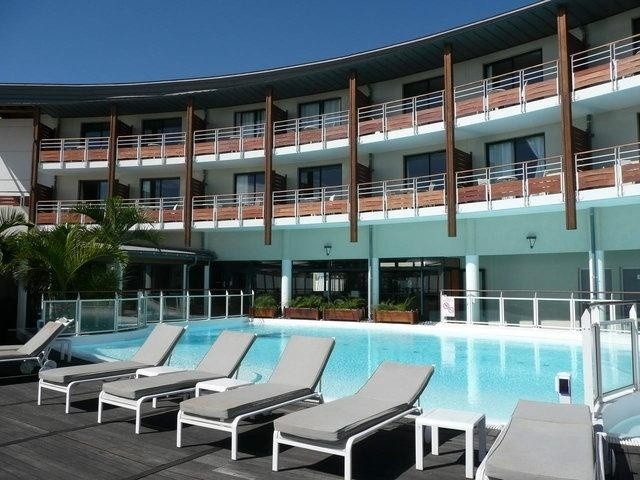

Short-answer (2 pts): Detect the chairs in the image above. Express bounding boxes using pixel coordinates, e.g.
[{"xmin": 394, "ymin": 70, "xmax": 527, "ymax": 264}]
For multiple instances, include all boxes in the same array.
[
  {"xmin": 36, "ymin": 318, "xmax": 72, "ymax": 365},
  {"xmin": 174, "ymin": 333, "xmax": 338, "ymax": 461},
  {"xmin": 33, "ymin": 321, "xmax": 188, "ymax": 415},
  {"xmin": 0, "ymin": 314, "xmax": 75, "ymax": 377},
  {"xmin": 269, "ymin": 357, "xmax": 436, "ymax": 480},
  {"xmin": 93, "ymin": 329, "xmax": 258, "ymax": 440},
  {"xmin": 474, "ymin": 397, "xmax": 609, "ymax": 480}
]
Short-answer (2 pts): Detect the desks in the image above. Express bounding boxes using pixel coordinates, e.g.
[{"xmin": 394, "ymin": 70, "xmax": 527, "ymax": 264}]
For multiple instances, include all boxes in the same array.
[
  {"xmin": 135, "ymin": 366, "xmax": 189, "ymax": 409},
  {"xmin": 411, "ymin": 405, "xmax": 489, "ymax": 480},
  {"xmin": 192, "ymin": 377, "xmax": 256, "ymax": 424}
]
